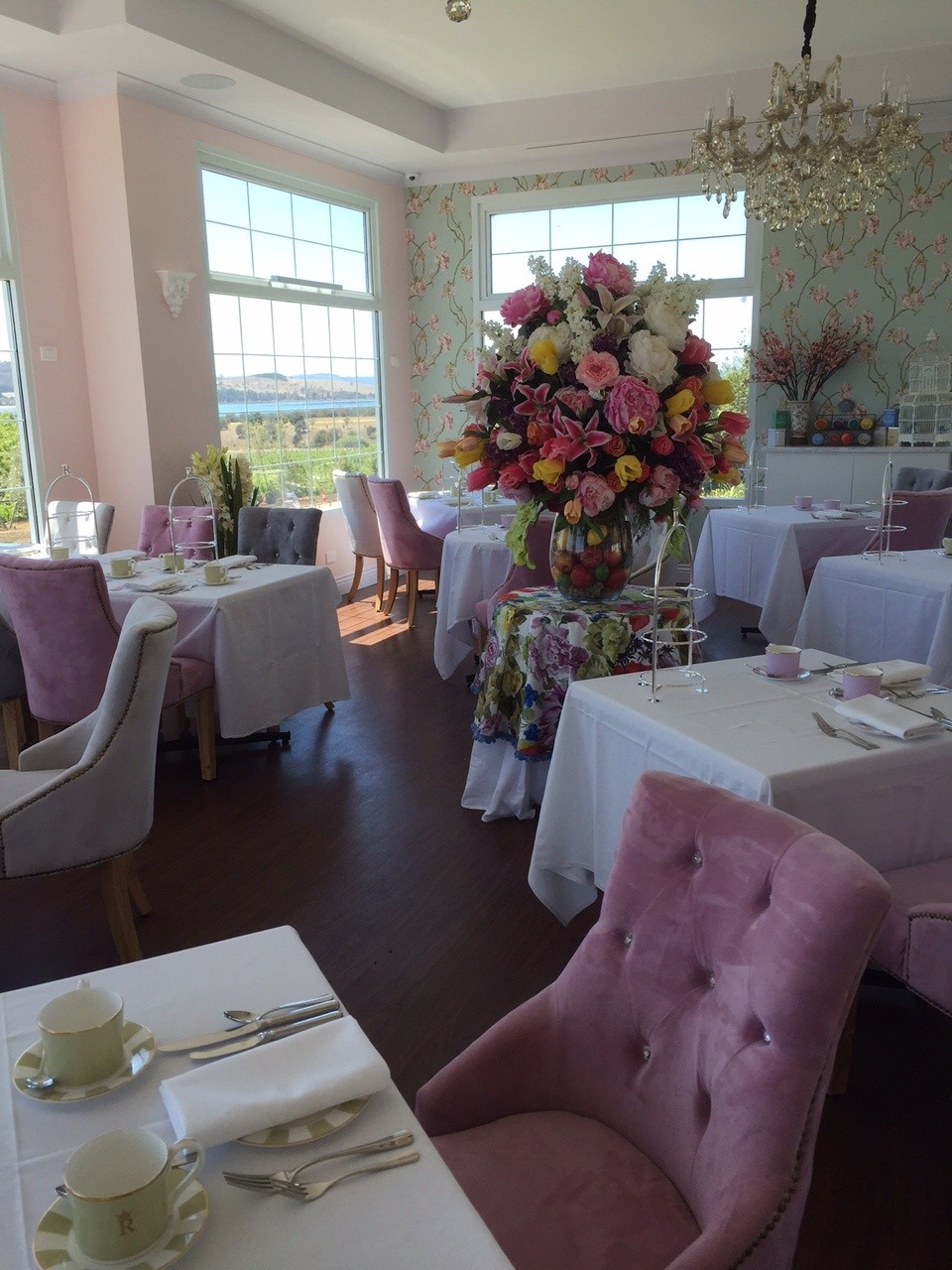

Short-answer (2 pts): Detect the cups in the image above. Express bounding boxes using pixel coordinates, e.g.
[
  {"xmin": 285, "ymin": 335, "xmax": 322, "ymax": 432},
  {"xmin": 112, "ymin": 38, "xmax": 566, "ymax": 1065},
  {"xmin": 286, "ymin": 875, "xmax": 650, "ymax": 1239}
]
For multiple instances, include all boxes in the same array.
[
  {"xmin": 61, "ymin": 1126, "xmax": 208, "ymax": 1263},
  {"xmin": 35, "ymin": 980, "xmax": 124, "ymax": 1086},
  {"xmin": 452, "ymin": 487, "xmax": 517, "ymax": 526},
  {"xmin": 942, "ymin": 538, "xmax": 952, "ymax": 554},
  {"xmin": 794, "ymin": 496, "xmax": 841, "ymax": 510},
  {"xmin": 50, "ymin": 547, "xmax": 228, "ymax": 583},
  {"xmin": 842, "ymin": 667, "xmax": 883, "ymax": 700},
  {"xmin": 764, "ymin": 643, "xmax": 802, "ymax": 678}
]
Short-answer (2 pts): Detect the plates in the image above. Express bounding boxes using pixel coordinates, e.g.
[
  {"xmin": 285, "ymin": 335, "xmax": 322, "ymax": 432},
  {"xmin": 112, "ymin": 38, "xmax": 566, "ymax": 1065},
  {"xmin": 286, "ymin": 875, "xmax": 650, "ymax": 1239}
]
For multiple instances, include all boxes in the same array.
[
  {"xmin": 232, "ymin": 1094, "xmax": 372, "ymax": 1148},
  {"xmin": 828, "ymin": 688, "xmax": 890, "ymax": 701},
  {"xmin": 936, "ymin": 551, "xmax": 952, "ymax": 558},
  {"xmin": 12, "ymin": 1018, "xmax": 155, "ymax": 1101},
  {"xmin": 750, "ymin": 664, "xmax": 811, "ymax": 681},
  {"xmin": 32, "ymin": 1166, "xmax": 208, "ymax": 1270}
]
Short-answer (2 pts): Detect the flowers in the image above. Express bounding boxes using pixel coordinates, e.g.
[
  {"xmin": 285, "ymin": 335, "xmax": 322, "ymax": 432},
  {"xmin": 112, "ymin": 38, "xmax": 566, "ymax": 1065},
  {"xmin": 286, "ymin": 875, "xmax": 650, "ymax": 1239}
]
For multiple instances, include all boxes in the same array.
[
  {"xmin": 737, "ymin": 312, "xmax": 877, "ymax": 399},
  {"xmin": 432, "ymin": 246, "xmax": 751, "ymax": 572},
  {"xmin": 186, "ymin": 446, "xmax": 263, "ymax": 556}
]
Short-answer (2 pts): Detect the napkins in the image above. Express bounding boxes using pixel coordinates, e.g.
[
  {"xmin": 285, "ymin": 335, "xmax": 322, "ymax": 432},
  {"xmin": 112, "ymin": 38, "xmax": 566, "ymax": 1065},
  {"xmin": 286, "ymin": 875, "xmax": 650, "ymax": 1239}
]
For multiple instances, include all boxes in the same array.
[
  {"xmin": 206, "ymin": 554, "xmax": 258, "ymax": 567},
  {"xmin": 826, "ymin": 658, "xmax": 933, "ymax": 685},
  {"xmin": 128, "ymin": 572, "xmax": 187, "ymax": 591},
  {"xmin": 815, "ymin": 508, "xmax": 859, "ymax": 519},
  {"xmin": 156, "ymin": 1013, "xmax": 392, "ymax": 1152},
  {"xmin": 833, "ymin": 695, "xmax": 948, "ymax": 741},
  {"xmin": 99, "ymin": 550, "xmax": 145, "ymax": 564}
]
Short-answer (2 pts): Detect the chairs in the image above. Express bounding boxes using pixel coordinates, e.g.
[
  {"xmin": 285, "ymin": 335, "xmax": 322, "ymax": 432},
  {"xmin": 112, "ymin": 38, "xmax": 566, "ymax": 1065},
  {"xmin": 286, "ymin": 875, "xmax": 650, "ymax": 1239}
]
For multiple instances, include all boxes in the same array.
[
  {"xmin": 0, "ymin": 504, "xmax": 324, "ymax": 964},
  {"xmin": 332, "ymin": 467, "xmax": 952, "ymax": 1269}
]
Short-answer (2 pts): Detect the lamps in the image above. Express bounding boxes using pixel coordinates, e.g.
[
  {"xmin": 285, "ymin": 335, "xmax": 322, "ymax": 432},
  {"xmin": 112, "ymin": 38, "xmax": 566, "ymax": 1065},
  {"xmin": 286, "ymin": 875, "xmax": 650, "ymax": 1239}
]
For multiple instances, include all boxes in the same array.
[{"xmin": 689, "ymin": 0, "xmax": 926, "ymax": 254}]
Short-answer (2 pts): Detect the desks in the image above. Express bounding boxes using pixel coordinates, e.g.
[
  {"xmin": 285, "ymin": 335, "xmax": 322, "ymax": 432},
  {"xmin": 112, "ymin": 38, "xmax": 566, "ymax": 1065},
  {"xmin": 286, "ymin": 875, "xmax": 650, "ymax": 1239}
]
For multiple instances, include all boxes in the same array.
[
  {"xmin": 458, "ymin": 585, "xmax": 707, "ymax": 821},
  {"xmin": 0, "ymin": 544, "xmax": 352, "ymax": 749},
  {"xmin": 760, "ymin": 446, "xmax": 952, "ymax": 508},
  {"xmin": 0, "ymin": 920, "xmax": 521, "ymax": 1270},
  {"xmin": 404, "ymin": 489, "xmax": 519, "ymax": 540},
  {"xmin": 692, "ymin": 504, "xmax": 880, "ymax": 645},
  {"xmin": 793, "ymin": 548, "xmax": 951, "ymax": 689},
  {"xmin": 432, "ymin": 523, "xmax": 512, "ymax": 683},
  {"xmin": 522, "ymin": 649, "xmax": 952, "ymax": 930}
]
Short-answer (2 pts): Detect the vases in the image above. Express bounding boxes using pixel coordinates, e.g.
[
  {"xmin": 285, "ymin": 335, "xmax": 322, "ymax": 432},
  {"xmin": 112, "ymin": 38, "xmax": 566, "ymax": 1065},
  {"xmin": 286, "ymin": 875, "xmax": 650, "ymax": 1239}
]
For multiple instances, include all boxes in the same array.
[
  {"xmin": 550, "ymin": 501, "xmax": 633, "ymax": 600},
  {"xmin": 786, "ymin": 400, "xmax": 811, "ymax": 444}
]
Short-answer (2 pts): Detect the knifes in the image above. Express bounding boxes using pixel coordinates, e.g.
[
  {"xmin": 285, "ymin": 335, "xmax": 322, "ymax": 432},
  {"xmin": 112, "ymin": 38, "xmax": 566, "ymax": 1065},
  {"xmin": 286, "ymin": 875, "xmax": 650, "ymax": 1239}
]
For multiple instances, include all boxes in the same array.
[
  {"xmin": 189, "ymin": 1010, "xmax": 344, "ymax": 1059},
  {"xmin": 155, "ymin": 998, "xmax": 340, "ymax": 1053}
]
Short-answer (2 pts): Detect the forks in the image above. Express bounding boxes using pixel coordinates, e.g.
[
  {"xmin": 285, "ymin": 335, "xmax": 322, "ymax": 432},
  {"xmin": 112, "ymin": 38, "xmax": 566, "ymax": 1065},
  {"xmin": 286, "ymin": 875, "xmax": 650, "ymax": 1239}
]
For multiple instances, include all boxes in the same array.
[
  {"xmin": 268, "ymin": 1152, "xmax": 420, "ymax": 1201},
  {"xmin": 886, "ymin": 687, "xmax": 952, "ymax": 699},
  {"xmin": 221, "ymin": 1129, "xmax": 415, "ymax": 1193},
  {"xmin": 810, "ymin": 711, "xmax": 880, "ymax": 750}
]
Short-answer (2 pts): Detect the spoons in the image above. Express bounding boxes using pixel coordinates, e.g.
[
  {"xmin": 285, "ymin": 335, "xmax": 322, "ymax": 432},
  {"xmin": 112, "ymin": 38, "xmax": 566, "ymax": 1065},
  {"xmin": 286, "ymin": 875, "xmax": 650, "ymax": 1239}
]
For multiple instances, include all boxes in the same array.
[
  {"xmin": 930, "ymin": 706, "xmax": 952, "ymax": 722},
  {"xmin": 222, "ymin": 993, "xmax": 334, "ymax": 1024},
  {"xmin": 25, "ymin": 1047, "xmax": 53, "ymax": 1089},
  {"xmin": 55, "ymin": 1150, "xmax": 198, "ymax": 1198}
]
